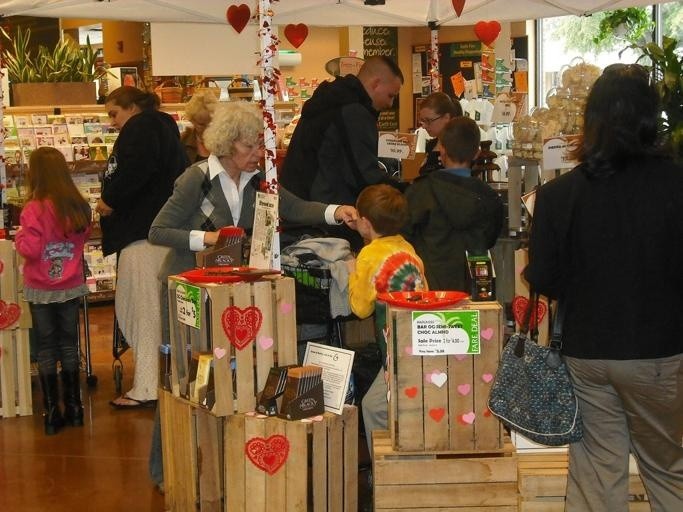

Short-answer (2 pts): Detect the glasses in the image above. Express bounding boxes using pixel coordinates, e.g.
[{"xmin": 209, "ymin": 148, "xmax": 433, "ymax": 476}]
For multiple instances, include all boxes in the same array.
[{"xmin": 417, "ymin": 113, "xmax": 446, "ymax": 127}]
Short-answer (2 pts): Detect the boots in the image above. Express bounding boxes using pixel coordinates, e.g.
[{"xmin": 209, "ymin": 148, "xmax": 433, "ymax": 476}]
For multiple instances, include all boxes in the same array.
[
  {"xmin": 39, "ymin": 372, "xmax": 66, "ymax": 437},
  {"xmin": 61, "ymin": 368, "xmax": 86, "ymax": 427}
]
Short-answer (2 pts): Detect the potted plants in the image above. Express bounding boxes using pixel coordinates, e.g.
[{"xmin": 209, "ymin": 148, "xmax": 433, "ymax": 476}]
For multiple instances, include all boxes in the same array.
[
  {"xmin": 0, "ymin": 22, "xmax": 118, "ymax": 107},
  {"xmin": 593, "ymin": 6, "xmax": 656, "ymax": 46}
]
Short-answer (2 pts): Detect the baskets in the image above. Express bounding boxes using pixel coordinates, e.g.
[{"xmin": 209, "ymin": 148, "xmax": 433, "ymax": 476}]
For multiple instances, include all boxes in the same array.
[{"xmin": 278, "ymin": 257, "xmax": 363, "ymax": 325}]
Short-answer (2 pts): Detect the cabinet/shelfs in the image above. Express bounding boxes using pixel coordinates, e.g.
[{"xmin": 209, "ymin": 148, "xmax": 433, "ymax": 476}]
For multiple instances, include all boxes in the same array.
[{"xmin": 5, "ymin": 156, "xmax": 113, "ymax": 304}]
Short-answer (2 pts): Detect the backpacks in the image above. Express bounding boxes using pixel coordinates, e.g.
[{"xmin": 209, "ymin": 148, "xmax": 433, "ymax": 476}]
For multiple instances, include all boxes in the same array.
[{"xmin": 484, "ymin": 330, "xmax": 585, "ymax": 448}]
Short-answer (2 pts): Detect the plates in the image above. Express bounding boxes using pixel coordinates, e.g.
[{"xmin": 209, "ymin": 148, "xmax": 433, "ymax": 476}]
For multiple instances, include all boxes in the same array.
[
  {"xmin": 377, "ymin": 291, "xmax": 471, "ymax": 310},
  {"xmin": 177, "ymin": 267, "xmax": 262, "ymax": 282}
]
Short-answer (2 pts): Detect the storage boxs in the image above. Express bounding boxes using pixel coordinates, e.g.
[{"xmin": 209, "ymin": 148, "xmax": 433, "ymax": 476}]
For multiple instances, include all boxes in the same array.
[
  {"xmin": 170, "ymin": 274, "xmax": 299, "ymax": 416},
  {"xmin": 223, "ymin": 405, "xmax": 358, "ymax": 511},
  {"xmin": 371, "ymin": 430, "xmax": 517, "ymax": 512},
  {"xmin": 519, "ymin": 475, "xmax": 652, "ymax": 512},
  {"xmin": 159, "ymin": 388, "xmax": 223, "ymax": 512},
  {"xmin": 386, "ymin": 301, "xmax": 503, "ymax": 455}
]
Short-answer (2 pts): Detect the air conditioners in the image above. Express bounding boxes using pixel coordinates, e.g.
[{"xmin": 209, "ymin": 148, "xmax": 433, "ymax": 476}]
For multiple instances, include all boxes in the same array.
[{"xmin": 277, "ymin": 50, "xmax": 302, "ymax": 68}]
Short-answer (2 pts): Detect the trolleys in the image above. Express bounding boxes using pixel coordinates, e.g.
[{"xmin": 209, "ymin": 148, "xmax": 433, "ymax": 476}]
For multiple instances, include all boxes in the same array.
[
  {"xmin": 111, "ymin": 250, "xmax": 131, "ymax": 393},
  {"xmin": 29, "ymin": 250, "xmax": 97, "ymax": 389},
  {"xmin": 270, "ymin": 217, "xmax": 379, "ymax": 493}
]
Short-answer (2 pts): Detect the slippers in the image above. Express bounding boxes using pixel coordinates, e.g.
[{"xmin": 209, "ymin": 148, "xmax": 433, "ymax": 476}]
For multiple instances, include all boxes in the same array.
[{"xmin": 107, "ymin": 393, "xmax": 157, "ymax": 410}]
